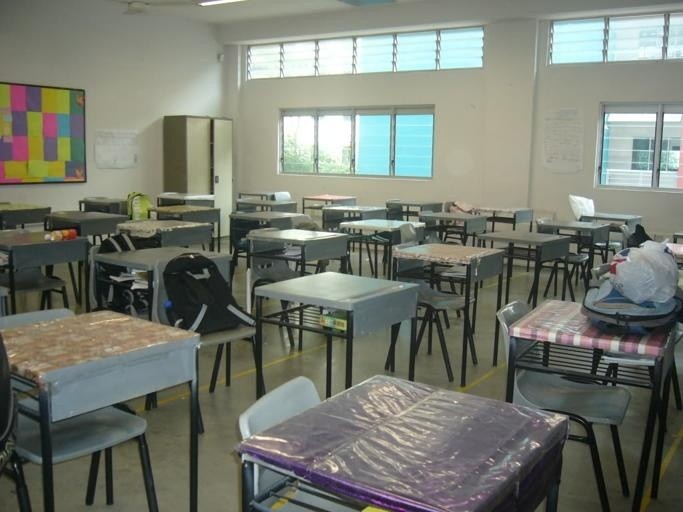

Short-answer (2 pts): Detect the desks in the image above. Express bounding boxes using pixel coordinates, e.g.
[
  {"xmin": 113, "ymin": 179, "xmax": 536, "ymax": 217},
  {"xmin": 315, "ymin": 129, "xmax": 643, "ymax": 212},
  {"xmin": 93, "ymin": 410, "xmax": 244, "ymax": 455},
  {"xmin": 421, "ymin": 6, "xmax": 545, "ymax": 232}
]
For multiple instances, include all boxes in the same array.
[
  {"xmin": 397, "ymin": 242, "xmax": 504, "ymax": 386},
  {"xmin": 94, "ymin": 244, "xmax": 235, "ymax": 325},
  {"xmin": 236, "ymin": 374, "xmax": 568, "ymax": 511},
  {"xmin": 251, "ymin": 272, "xmax": 420, "ymax": 400},
  {"xmin": 502, "ymin": 298, "xmax": 681, "ymax": 510},
  {"xmin": 1, "ymin": 311, "xmax": 202, "ymax": 510}
]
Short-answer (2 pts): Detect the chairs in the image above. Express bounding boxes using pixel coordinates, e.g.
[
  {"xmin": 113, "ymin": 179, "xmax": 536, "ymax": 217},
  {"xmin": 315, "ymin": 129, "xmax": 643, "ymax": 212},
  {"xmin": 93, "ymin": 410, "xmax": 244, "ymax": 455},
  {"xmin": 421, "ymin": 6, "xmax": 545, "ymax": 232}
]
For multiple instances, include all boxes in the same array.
[
  {"xmin": 497, "ymin": 301, "xmax": 631, "ymax": 510},
  {"xmin": 162, "ymin": 252, "xmax": 266, "ymax": 434},
  {"xmin": 0, "ymin": 307, "xmax": 160, "ymax": 511},
  {"xmin": 1, "ymin": 238, "xmax": 90, "ymax": 315},
  {"xmin": 1, "ymin": 191, "xmax": 682, "ymax": 274},
  {"xmin": 383, "ymin": 241, "xmax": 478, "ymax": 381},
  {"xmin": 238, "ymin": 375, "xmax": 373, "ymax": 511}
]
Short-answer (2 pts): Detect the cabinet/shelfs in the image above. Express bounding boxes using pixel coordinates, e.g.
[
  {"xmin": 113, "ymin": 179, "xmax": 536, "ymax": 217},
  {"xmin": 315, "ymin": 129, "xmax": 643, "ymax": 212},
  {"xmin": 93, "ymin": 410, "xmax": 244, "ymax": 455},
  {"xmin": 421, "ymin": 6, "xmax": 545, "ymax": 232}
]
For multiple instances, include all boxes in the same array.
[{"xmin": 162, "ymin": 116, "xmax": 233, "ymax": 241}]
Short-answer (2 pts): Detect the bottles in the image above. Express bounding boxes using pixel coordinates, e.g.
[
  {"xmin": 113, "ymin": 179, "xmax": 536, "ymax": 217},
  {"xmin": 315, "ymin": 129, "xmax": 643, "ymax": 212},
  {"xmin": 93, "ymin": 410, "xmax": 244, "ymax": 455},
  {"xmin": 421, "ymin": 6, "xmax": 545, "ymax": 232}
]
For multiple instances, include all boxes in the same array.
[{"xmin": 42, "ymin": 227, "xmax": 78, "ymax": 242}]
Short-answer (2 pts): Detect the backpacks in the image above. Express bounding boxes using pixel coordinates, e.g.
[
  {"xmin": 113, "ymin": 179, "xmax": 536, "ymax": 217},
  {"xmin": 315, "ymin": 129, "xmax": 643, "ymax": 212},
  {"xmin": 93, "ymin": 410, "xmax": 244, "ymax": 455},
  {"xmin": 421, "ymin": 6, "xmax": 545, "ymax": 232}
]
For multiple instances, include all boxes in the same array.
[
  {"xmin": 96, "ymin": 233, "xmax": 162, "ymax": 315},
  {"xmin": 621, "ymin": 224, "xmax": 652, "ymax": 248},
  {"xmin": 164, "ymin": 252, "xmax": 256, "ymax": 335},
  {"xmin": 580, "ymin": 285, "xmax": 682, "ymax": 335},
  {"xmin": 449, "ymin": 201, "xmax": 473, "ymax": 226},
  {"xmin": 127, "ymin": 191, "xmax": 158, "ymax": 221}
]
